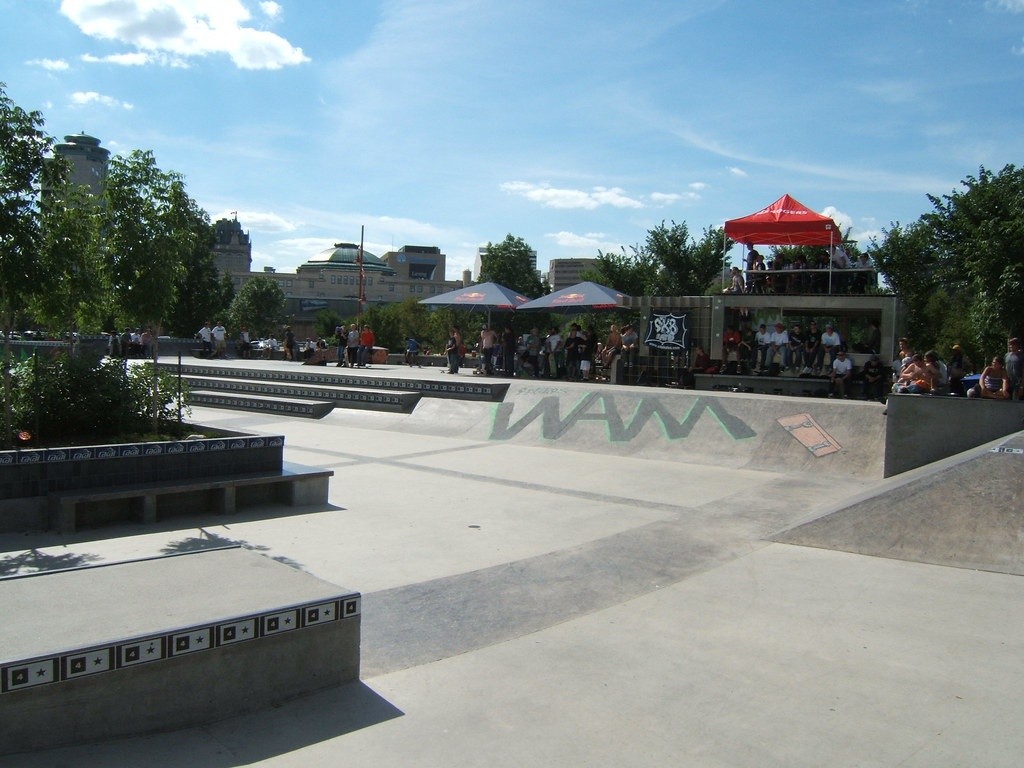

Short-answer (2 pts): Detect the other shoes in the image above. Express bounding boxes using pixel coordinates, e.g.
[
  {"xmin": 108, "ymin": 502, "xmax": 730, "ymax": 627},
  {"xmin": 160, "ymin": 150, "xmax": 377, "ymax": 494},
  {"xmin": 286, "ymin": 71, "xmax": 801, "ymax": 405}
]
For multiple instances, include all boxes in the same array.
[
  {"xmin": 629, "ymin": 362, "xmax": 633, "ymax": 367},
  {"xmin": 599, "ymin": 367, "xmax": 608, "ymax": 373},
  {"xmin": 755, "ymin": 364, "xmax": 833, "ymax": 375},
  {"xmin": 624, "ymin": 362, "xmax": 627, "ymax": 367},
  {"xmin": 720, "ymin": 365, "xmax": 727, "ymax": 373},
  {"xmin": 736, "ymin": 364, "xmax": 741, "ymax": 373}
]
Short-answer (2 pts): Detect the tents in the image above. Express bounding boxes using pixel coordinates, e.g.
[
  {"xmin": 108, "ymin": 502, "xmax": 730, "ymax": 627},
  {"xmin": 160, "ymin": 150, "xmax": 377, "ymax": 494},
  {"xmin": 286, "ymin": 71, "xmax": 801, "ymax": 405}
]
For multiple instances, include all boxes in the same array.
[{"xmin": 723, "ymin": 195, "xmax": 845, "ymax": 294}]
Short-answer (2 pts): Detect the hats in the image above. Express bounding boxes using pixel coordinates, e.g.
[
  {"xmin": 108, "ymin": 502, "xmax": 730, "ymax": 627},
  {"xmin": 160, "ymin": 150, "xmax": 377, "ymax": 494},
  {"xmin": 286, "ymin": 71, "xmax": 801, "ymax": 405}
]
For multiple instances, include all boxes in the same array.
[
  {"xmin": 900, "ymin": 357, "xmax": 911, "ymax": 368},
  {"xmin": 825, "ymin": 325, "xmax": 832, "ymax": 329},
  {"xmin": 953, "ymin": 345, "xmax": 961, "ymax": 350},
  {"xmin": 774, "ymin": 323, "xmax": 782, "ymax": 329}
]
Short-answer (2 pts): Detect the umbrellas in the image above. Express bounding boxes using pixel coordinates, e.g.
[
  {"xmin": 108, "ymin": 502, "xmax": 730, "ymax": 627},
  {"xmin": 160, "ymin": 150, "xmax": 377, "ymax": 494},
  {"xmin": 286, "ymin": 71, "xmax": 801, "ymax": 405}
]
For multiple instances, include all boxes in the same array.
[
  {"xmin": 477, "ymin": 323, "xmax": 638, "ymax": 382},
  {"xmin": 515, "ymin": 281, "xmax": 642, "ymax": 332},
  {"xmin": 417, "ymin": 282, "xmax": 531, "ymax": 329}
]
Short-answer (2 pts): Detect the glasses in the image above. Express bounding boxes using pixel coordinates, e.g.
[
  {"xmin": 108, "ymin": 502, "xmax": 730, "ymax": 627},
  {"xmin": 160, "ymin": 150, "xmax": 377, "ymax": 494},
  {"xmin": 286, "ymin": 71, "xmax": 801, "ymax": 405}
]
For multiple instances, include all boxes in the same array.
[{"xmin": 836, "ymin": 354, "xmax": 844, "ymax": 357}]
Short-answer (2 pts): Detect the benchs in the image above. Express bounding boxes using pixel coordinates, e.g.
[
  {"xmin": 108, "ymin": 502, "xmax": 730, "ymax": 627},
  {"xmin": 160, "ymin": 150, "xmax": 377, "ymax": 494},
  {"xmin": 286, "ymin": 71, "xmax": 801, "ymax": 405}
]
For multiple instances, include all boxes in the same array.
[{"xmin": 191, "ymin": 348, "xmax": 214, "ymax": 358}]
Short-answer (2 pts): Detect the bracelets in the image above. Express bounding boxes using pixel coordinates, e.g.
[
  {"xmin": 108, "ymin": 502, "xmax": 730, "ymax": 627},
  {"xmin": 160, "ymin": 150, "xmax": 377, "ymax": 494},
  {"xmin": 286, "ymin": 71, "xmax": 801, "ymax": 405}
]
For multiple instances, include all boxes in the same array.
[{"xmin": 1020, "ymin": 387, "xmax": 1023, "ymax": 388}]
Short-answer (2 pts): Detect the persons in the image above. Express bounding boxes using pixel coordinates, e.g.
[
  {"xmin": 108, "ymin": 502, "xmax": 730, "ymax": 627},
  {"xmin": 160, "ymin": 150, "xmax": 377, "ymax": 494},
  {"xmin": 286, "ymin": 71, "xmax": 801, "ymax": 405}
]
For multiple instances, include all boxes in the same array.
[
  {"xmin": 261, "ymin": 325, "xmax": 327, "ymax": 365},
  {"xmin": 443, "ymin": 326, "xmax": 467, "ymax": 374},
  {"xmin": 241, "ymin": 326, "xmax": 252, "ymax": 358},
  {"xmin": 967, "ymin": 338, "xmax": 1024, "ymax": 399},
  {"xmin": 109, "ymin": 328, "xmax": 154, "ymax": 359},
  {"xmin": 863, "ymin": 356, "xmax": 885, "ymax": 403},
  {"xmin": 195, "ymin": 321, "xmax": 229, "ymax": 358},
  {"xmin": 679, "ymin": 345, "xmax": 710, "ymax": 389},
  {"xmin": 335, "ymin": 324, "xmax": 374, "ymax": 367},
  {"xmin": 720, "ymin": 321, "xmax": 852, "ymax": 399},
  {"xmin": 852, "ymin": 319, "xmax": 881, "ymax": 354},
  {"xmin": 849, "ymin": 253, "xmax": 873, "ymax": 294},
  {"xmin": 723, "ymin": 241, "xmax": 850, "ymax": 294},
  {"xmin": 404, "ymin": 337, "xmax": 422, "ymax": 368},
  {"xmin": 882, "ymin": 338, "xmax": 966, "ymax": 415},
  {"xmin": 36, "ymin": 330, "xmax": 41, "ymax": 340},
  {"xmin": 424, "ymin": 346, "xmax": 433, "ymax": 355}
]
